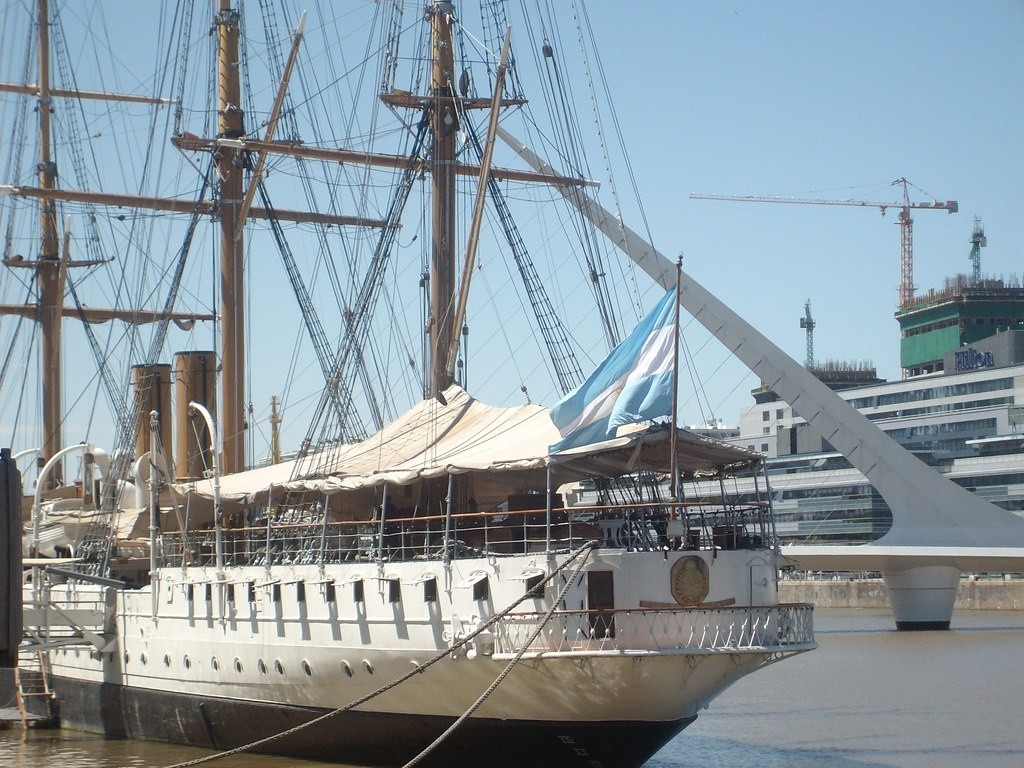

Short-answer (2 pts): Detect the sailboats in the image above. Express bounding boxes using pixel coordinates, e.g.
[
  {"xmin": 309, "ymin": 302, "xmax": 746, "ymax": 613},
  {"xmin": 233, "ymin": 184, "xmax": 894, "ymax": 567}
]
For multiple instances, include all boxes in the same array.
[{"xmin": 0, "ymin": 0, "xmax": 819, "ymax": 768}]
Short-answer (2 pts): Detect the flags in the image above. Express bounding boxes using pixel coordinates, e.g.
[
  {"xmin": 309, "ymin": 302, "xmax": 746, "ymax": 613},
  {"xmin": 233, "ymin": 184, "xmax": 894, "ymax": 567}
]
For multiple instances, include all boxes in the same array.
[{"xmin": 546, "ymin": 284, "xmax": 676, "ymax": 452}]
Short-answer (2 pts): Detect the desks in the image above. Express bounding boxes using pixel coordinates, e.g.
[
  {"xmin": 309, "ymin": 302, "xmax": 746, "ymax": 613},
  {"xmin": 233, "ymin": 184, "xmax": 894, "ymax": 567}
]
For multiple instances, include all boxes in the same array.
[
  {"xmin": 685, "ymin": 529, "xmax": 701, "ymax": 550},
  {"xmin": 711, "ymin": 525, "xmax": 744, "ymax": 549}
]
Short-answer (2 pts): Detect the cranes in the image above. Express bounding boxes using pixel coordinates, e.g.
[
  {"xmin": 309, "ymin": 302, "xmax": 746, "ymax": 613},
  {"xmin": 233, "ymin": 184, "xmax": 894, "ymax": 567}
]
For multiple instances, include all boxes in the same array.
[{"xmin": 689, "ymin": 177, "xmax": 958, "ymax": 380}]
